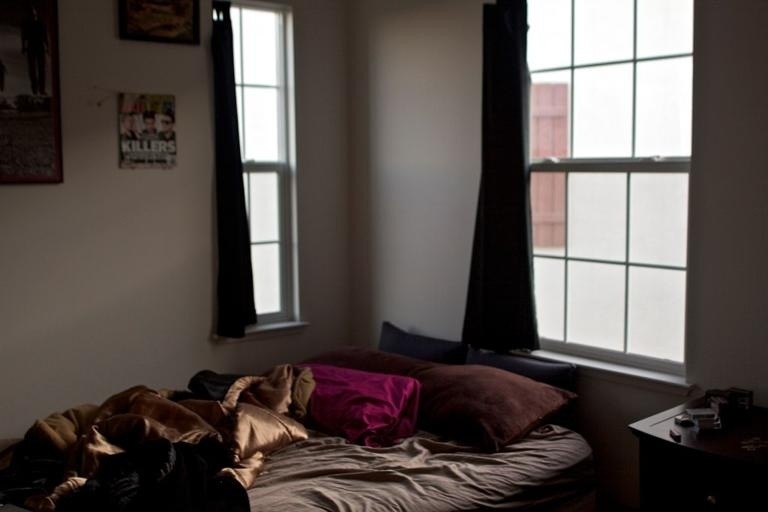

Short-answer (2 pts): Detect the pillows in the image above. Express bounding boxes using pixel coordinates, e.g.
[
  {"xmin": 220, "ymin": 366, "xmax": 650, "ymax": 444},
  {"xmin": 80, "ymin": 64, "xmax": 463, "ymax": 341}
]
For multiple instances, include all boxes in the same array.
[{"xmin": 288, "ymin": 343, "xmax": 579, "ymax": 454}]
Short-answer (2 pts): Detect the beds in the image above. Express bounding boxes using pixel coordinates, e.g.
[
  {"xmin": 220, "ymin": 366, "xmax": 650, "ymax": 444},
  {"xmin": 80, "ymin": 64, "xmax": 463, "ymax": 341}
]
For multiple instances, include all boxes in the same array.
[{"xmin": 245, "ymin": 320, "xmax": 591, "ymax": 512}]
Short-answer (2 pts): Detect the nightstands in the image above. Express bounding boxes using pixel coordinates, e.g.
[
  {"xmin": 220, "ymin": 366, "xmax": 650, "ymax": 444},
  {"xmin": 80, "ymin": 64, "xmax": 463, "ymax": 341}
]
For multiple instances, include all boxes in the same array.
[{"xmin": 626, "ymin": 397, "xmax": 768, "ymax": 511}]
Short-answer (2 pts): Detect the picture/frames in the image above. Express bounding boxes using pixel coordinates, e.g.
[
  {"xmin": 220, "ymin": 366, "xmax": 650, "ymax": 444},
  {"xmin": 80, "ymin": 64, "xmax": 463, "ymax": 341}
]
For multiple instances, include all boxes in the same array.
[
  {"xmin": 114, "ymin": 1, "xmax": 202, "ymax": 45},
  {"xmin": 0, "ymin": 1, "xmax": 63, "ymax": 186}
]
